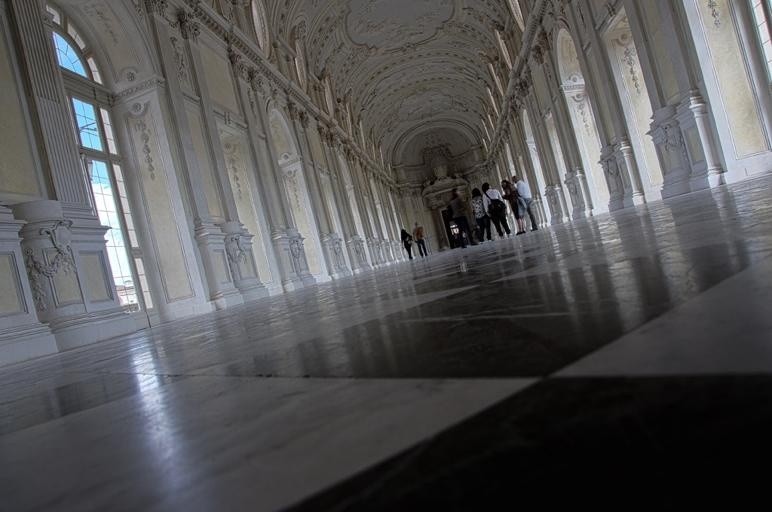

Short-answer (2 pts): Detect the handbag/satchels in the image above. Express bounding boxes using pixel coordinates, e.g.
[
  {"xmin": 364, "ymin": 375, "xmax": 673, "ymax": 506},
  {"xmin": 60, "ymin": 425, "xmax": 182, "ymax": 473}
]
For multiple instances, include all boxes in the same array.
[{"xmin": 491, "ymin": 199, "xmax": 507, "ymax": 212}]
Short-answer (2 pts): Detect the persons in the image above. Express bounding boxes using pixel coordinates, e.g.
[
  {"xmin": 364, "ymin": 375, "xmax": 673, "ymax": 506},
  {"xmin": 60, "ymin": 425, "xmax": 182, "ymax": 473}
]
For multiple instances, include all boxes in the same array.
[
  {"xmin": 401, "ymin": 229, "xmax": 413, "ymax": 259},
  {"xmin": 413, "ymin": 221, "xmax": 428, "ymax": 258},
  {"xmin": 448, "ymin": 188, "xmax": 479, "ymax": 248},
  {"xmin": 471, "ymin": 176, "xmax": 538, "ymax": 244}
]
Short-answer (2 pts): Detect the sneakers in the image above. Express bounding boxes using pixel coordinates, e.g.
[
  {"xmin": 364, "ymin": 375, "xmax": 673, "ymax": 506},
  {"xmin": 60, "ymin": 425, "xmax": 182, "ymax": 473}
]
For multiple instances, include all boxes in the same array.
[{"xmin": 462, "ymin": 227, "xmax": 539, "ymax": 248}]
